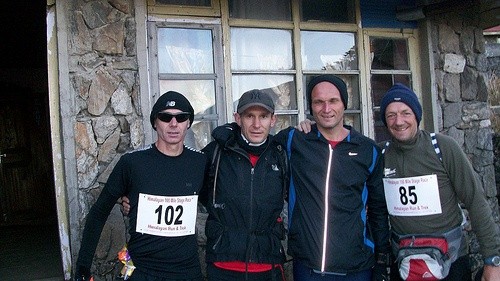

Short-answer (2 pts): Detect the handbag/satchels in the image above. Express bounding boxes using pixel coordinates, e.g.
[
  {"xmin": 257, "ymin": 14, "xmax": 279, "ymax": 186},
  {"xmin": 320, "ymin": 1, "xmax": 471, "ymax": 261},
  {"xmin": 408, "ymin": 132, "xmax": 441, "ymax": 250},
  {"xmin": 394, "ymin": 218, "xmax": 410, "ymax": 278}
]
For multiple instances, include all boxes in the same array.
[{"xmin": 388, "ymin": 226, "xmax": 469, "ymax": 281}]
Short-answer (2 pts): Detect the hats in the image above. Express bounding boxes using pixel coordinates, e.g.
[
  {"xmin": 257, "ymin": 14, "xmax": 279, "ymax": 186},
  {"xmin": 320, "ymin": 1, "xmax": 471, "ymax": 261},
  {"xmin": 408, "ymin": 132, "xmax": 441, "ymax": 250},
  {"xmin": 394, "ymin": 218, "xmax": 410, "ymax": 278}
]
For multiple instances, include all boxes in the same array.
[
  {"xmin": 380, "ymin": 83, "xmax": 422, "ymax": 127},
  {"xmin": 308, "ymin": 76, "xmax": 348, "ymax": 111},
  {"xmin": 238, "ymin": 89, "xmax": 275, "ymax": 113},
  {"xmin": 150, "ymin": 92, "xmax": 194, "ymax": 131}
]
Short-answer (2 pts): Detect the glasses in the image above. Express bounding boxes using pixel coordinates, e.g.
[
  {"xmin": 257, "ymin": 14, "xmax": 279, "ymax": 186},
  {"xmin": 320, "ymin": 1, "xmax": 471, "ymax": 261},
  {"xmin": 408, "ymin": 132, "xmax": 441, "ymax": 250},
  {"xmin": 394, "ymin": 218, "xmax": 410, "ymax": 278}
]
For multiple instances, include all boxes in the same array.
[{"xmin": 154, "ymin": 111, "xmax": 191, "ymax": 123}]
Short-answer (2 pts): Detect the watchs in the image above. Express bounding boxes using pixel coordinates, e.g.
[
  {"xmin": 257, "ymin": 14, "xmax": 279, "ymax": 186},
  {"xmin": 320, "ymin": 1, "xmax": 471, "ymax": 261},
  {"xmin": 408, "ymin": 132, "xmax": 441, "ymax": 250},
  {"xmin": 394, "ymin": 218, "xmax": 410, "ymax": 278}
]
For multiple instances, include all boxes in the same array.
[{"xmin": 484, "ymin": 256, "xmax": 500, "ymax": 267}]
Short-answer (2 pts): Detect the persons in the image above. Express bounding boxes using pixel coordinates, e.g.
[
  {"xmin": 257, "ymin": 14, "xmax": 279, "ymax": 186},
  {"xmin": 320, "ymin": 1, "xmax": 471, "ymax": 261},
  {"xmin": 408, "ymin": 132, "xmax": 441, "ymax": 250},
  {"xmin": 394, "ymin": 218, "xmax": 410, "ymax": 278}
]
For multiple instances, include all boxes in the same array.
[
  {"xmin": 293, "ymin": 83, "xmax": 500, "ymax": 281},
  {"xmin": 75, "ymin": 91, "xmax": 209, "ymax": 281},
  {"xmin": 273, "ymin": 76, "xmax": 389, "ymax": 281},
  {"xmin": 120, "ymin": 89, "xmax": 289, "ymax": 281}
]
